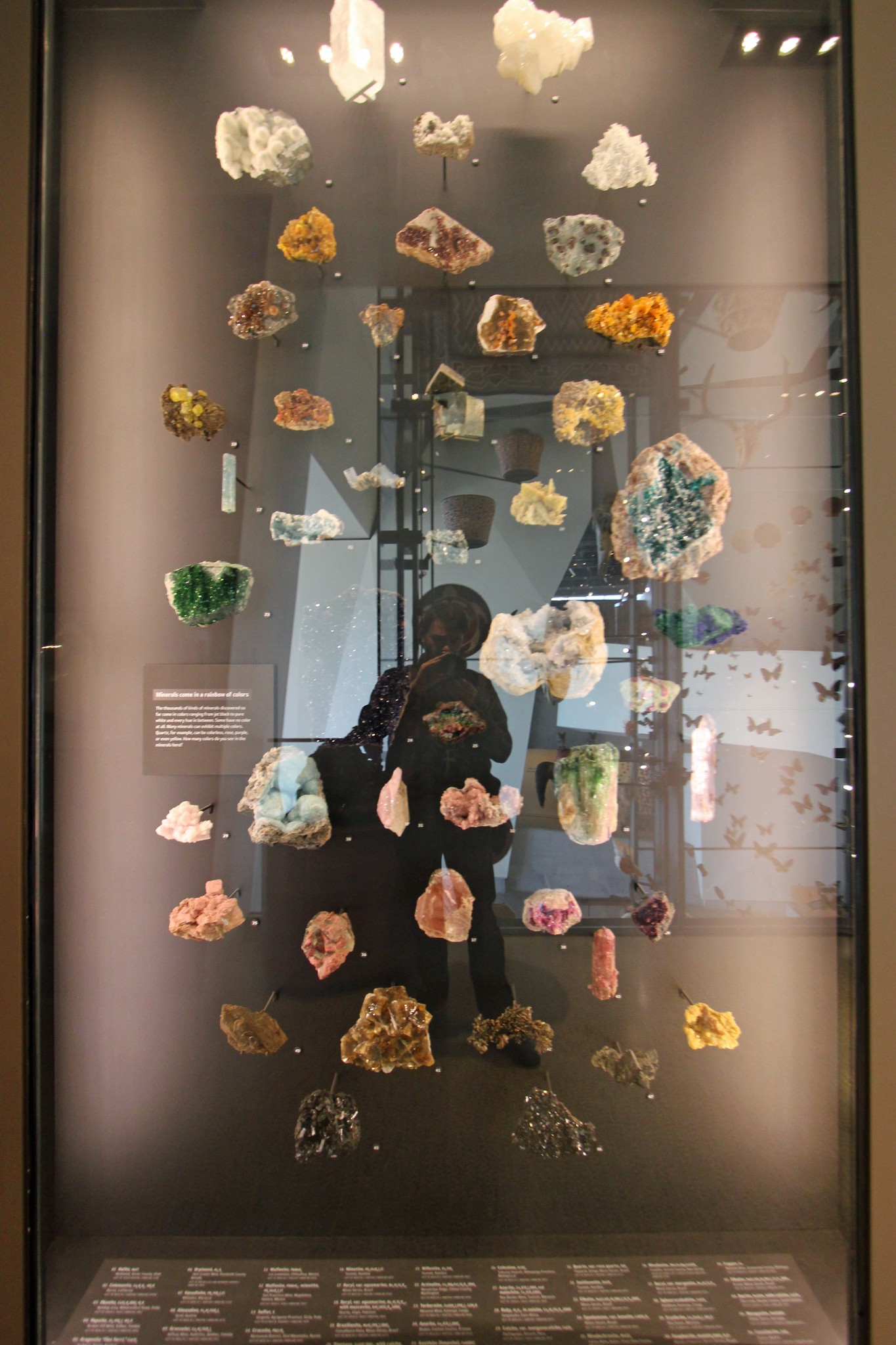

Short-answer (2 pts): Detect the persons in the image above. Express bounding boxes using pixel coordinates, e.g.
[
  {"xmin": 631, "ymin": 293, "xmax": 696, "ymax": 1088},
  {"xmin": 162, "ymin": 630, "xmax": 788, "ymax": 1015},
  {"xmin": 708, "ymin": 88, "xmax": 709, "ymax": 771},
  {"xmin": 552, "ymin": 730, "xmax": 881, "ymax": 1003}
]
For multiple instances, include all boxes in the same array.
[{"xmin": 329, "ymin": 600, "xmax": 539, "ymax": 1068}]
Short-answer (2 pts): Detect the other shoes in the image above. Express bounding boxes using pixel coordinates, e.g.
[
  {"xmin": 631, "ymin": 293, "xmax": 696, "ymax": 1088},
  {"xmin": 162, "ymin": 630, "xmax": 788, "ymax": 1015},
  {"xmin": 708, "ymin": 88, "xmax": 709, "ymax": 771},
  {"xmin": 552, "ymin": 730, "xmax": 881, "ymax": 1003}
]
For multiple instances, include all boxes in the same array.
[
  {"xmin": 427, "ymin": 1019, "xmax": 446, "ymax": 1038},
  {"xmin": 489, "ymin": 1032, "xmax": 540, "ymax": 1070}
]
